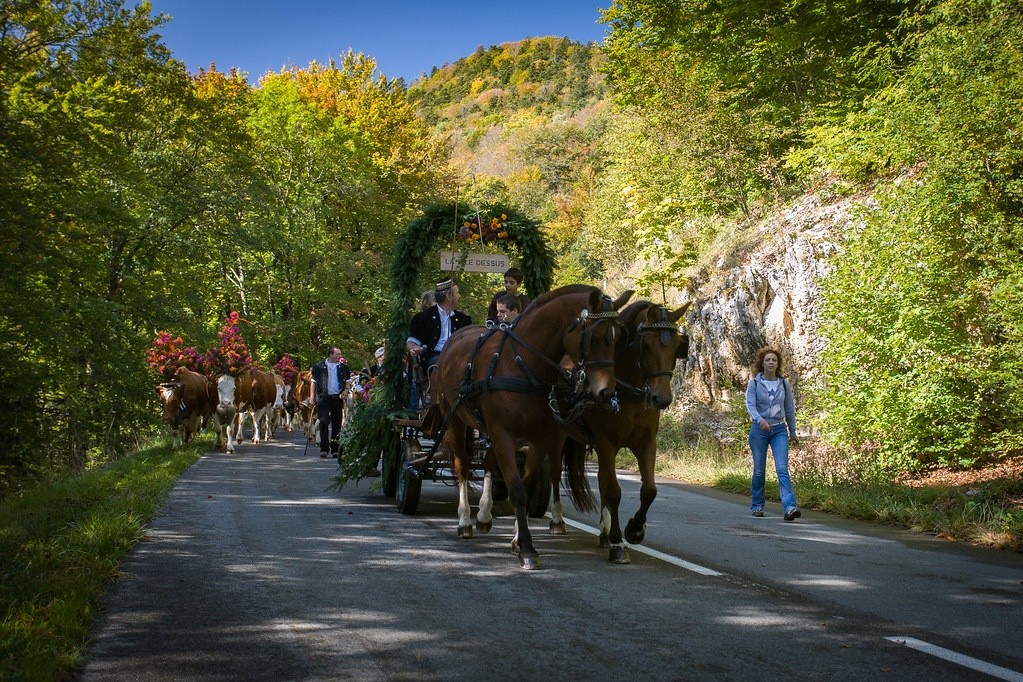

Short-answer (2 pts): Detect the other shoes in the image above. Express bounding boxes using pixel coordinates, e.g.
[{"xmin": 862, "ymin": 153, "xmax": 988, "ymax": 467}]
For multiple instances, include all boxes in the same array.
[
  {"xmin": 365, "ymin": 470, "xmax": 381, "ymax": 477},
  {"xmin": 320, "ymin": 452, "xmax": 328, "ymax": 458},
  {"xmin": 333, "ymin": 453, "xmax": 339, "ymax": 458}
]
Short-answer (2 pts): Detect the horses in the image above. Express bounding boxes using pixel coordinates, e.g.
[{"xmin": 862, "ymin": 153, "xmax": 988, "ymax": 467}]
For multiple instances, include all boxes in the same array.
[
  {"xmin": 429, "ymin": 283, "xmax": 639, "ymax": 570},
  {"xmin": 548, "ymin": 300, "xmax": 693, "ymax": 565}
]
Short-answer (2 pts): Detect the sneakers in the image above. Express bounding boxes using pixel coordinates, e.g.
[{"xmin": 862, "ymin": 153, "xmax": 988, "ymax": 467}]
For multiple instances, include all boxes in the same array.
[
  {"xmin": 752, "ymin": 510, "xmax": 764, "ymax": 517},
  {"xmin": 784, "ymin": 509, "xmax": 801, "ymax": 521}
]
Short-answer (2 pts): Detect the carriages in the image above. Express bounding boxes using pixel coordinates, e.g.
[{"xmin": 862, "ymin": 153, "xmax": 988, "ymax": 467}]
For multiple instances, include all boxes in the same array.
[{"xmin": 378, "ymin": 280, "xmax": 693, "ymax": 571}]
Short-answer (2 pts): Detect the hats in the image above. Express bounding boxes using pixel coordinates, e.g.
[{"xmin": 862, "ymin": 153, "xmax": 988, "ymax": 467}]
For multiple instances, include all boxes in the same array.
[
  {"xmin": 375, "ymin": 347, "xmax": 385, "ymax": 357},
  {"xmin": 436, "ymin": 277, "xmax": 456, "ymax": 291}
]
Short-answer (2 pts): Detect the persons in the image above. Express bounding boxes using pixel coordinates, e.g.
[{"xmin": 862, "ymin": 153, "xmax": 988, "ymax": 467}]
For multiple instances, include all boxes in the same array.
[
  {"xmin": 488, "ymin": 267, "xmax": 532, "ymax": 324},
  {"xmin": 745, "ymin": 348, "xmax": 802, "ymax": 520},
  {"xmin": 421, "ymin": 290, "xmax": 437, "ymax": 312},
  {"xmin": 496, "ymin": 294, "xmax": 522, "ymax": 325},
  {"xmin": 308, "ymin": 347, "xmax": 352, "ymax": 458},
  {"xmin": 359, "ymin": 346, "xmax": 385, "ymax": 387},
  {"xmin": 406, "ymin": 276, "xmax": 480, "ymax": 439}
]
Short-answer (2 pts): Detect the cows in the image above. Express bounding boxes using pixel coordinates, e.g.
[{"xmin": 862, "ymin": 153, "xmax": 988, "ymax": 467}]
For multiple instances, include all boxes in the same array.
[
  {"xmin": 208, "ymin": 370, "xmax": 322, "ymax": 459},
  {"xmin": 154, "ymin": 366, "xmax": 213, "ymax": 451}
]
